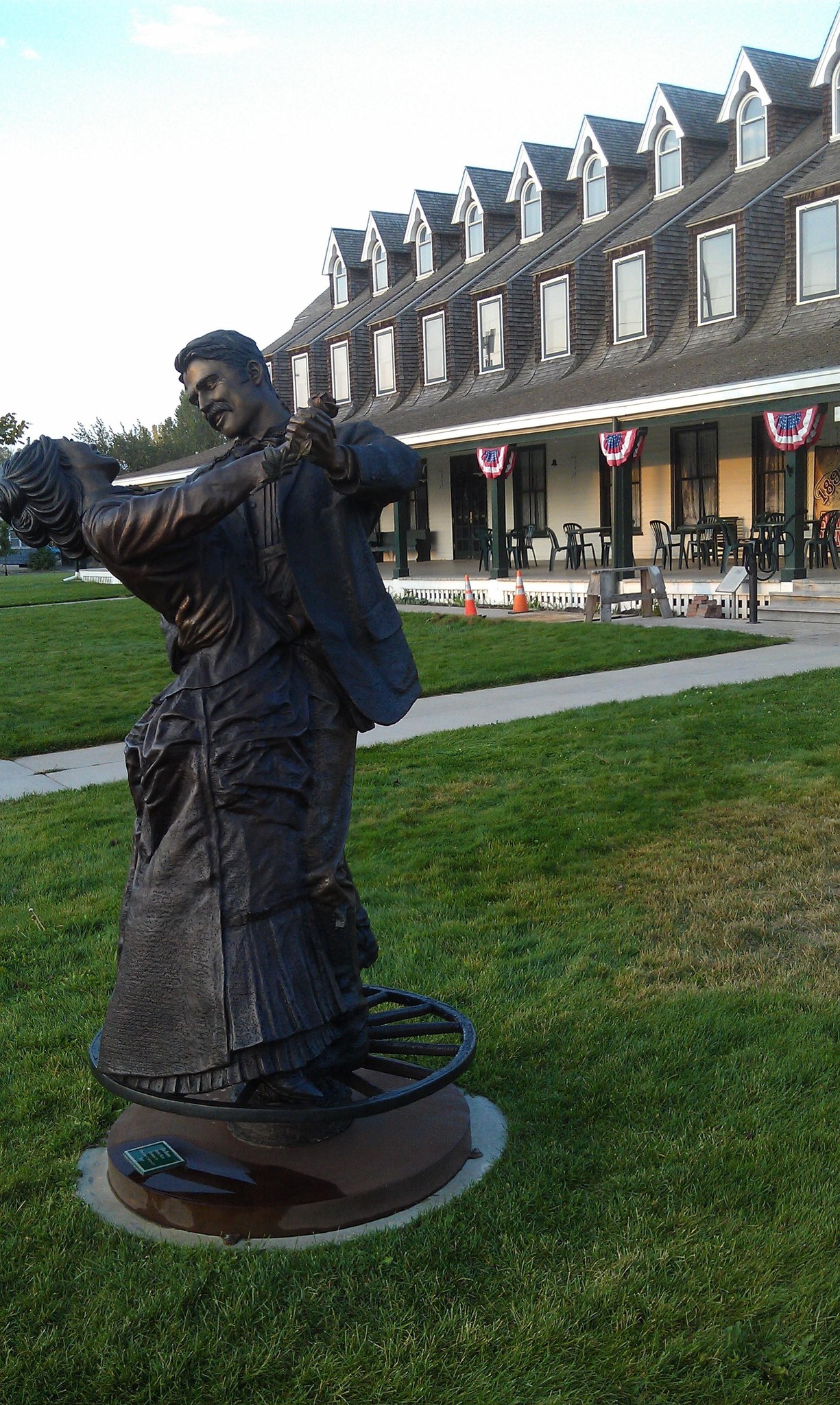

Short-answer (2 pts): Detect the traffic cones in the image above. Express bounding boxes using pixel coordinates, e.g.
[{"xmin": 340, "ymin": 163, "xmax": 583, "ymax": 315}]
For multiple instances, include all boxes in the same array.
[
  {"xmin": 463, "ymin": 574, "xmax": 487, "ymax": 619},
  {"xmin": 508, "ymin": 570, "xmax": 538, "ymax": 614}
]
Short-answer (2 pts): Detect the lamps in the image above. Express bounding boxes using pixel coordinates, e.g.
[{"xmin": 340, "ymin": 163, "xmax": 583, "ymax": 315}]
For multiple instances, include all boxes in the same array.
[
  {"xmin": 472, "ymin": 467, "xmax": 483, "ymax": 476},
  {"xmin": 419, "ymin": 461, "xmax": 432, "ymax": 482}
]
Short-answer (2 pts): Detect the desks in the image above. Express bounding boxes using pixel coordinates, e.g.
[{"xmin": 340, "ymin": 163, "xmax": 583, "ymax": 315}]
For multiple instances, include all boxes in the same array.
[
  {"xmin": 677, "ymin": 522, "xmax": 734, "ymax": 570},
  {"xmin": 485, "ymin": 533, "xmax": 518, "ymax": 570},
  {"xmin": 754, "ymin": 520, "xmax": 824, "ymax": 570},
  {"xmin": 566, "ymin": 526, "xmax": 611, "ymax": 569}
]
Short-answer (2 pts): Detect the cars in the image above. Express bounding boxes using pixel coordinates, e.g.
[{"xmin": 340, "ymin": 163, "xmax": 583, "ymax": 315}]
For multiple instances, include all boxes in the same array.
[{"xmin": 46, "ymin": 545, "xmax": 62, "ymax": 560}]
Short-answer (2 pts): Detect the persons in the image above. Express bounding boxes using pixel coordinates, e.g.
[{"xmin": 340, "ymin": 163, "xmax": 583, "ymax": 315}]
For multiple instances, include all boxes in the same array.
[
  {"xmin": 160, "ymin": 330, "xmax": 422, "ymax": 1061},
  {"xmin": 0, "ymin": 391, "xmax": 347, "ymax": 1100}
]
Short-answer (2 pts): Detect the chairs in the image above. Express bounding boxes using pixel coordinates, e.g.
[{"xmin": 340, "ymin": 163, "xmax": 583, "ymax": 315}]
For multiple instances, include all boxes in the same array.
[
  {"xmin": 472, "ymin": 524, "xmax": 537, "ymax": 572},
  {"xmin": 650, "ymin": 508, "xmax": 840, "ymax": 574},
  {"xmin": 544, "ymin": 520, "xmax": 635, "ymax": 572}
]
